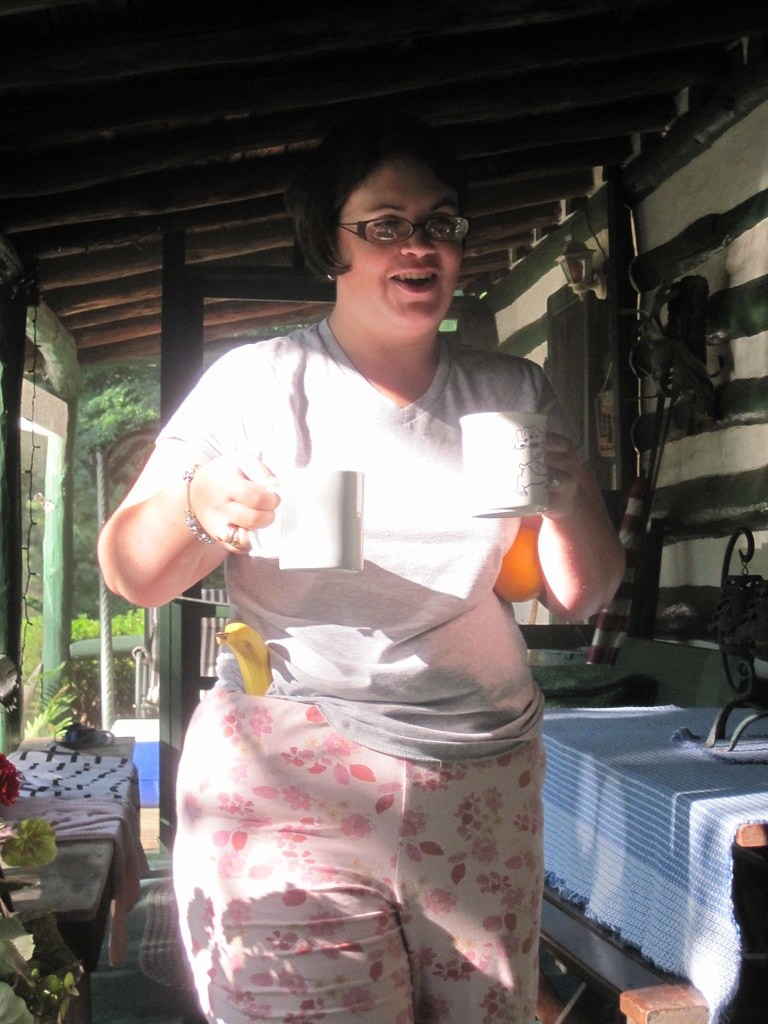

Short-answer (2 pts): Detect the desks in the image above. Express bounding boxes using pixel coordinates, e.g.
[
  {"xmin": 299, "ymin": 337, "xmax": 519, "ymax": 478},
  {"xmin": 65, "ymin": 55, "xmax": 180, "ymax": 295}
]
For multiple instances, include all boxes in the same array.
[
  {"xmin": 537, "ymin": 704, "xmax": 768, "ymax": 1024},
  {"xmin": 0, "ymin": 738, "xmax": 137, "ymax": 1024}
]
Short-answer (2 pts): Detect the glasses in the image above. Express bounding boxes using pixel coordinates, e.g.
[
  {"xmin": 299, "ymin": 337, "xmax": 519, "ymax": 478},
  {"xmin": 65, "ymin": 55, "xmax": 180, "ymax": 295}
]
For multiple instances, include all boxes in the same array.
[{"xmin": 336, "ymin": 214, "xmax": 468, "ymax": 246}]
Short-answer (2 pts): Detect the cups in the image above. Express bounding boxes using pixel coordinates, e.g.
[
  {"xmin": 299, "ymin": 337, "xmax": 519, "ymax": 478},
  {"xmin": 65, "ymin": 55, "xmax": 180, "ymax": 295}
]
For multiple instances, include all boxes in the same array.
[
  {"xmin": 460, "ymin": 409, "xmax": 546, "ymax": 519},
  {"xmin": 250, "ymin": 471, "xmax": 362, "ymax": 571}
]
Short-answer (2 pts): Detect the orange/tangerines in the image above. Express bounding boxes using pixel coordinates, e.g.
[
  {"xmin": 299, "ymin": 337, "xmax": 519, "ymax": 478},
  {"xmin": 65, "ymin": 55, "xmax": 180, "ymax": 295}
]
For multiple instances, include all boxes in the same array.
[{"xmin": 492, "ymin": 526, "xmax": 549, "ymax": 603}]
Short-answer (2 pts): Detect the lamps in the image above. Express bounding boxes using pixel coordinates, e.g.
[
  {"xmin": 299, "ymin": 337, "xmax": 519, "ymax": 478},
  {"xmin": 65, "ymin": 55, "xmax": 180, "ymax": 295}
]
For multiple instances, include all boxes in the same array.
[{"xmin": 554, "ymin": 242, "xmax": 608, "ymax": 301}]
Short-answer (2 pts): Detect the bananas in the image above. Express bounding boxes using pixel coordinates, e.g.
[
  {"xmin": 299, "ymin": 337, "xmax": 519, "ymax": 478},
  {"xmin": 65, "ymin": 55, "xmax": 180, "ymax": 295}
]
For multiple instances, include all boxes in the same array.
[{"xmin": 213, "ymin": 623, "xmax": 274, "ymax": 697}]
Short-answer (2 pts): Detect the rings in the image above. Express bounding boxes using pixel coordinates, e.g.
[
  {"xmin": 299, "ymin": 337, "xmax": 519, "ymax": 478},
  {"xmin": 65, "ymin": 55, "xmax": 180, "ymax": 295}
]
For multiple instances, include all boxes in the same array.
[
  {"xmin": 231, "ymin": 530, "xmax": 243, "ymax": 548},
  {"xmin": 97, "ymin": 118, "xmax": 627, "ymax": 1024},
  {"xmin": 548, "ymin": 468, "xmax": 563, "ymax": 490},
  {"xmin": 224, "ymin": 526, "xmax": 236, "ymax": 543}
]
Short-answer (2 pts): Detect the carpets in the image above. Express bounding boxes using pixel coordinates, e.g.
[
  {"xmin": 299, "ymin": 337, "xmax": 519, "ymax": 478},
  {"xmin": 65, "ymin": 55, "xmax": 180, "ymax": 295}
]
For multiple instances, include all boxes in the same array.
[{"xmin": 139, "ymin": 881, "xmax": 195, "ymax": 993}]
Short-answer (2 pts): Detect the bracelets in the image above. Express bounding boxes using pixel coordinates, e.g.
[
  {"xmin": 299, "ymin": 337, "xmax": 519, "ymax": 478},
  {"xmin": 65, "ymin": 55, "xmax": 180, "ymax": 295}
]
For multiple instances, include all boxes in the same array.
[{"xmin": 183, "ymin": 463, "xmax": 217, "ymax": 544}]
